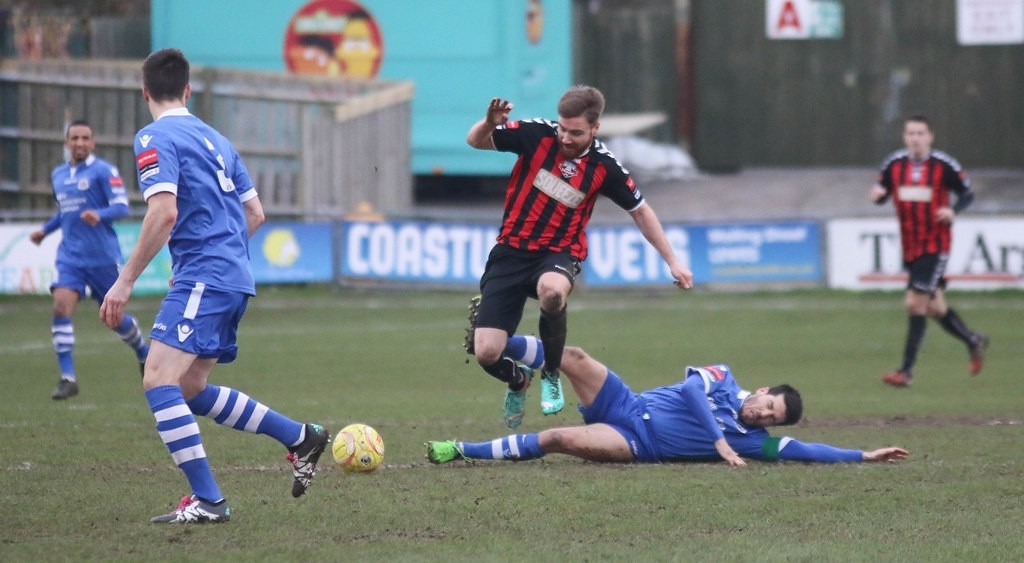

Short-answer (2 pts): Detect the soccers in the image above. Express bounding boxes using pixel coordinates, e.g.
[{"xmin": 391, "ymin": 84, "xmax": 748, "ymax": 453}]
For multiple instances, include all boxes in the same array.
[{"xmin": 331, "ymin": 419, "xmax": 384, "ymax": 475}]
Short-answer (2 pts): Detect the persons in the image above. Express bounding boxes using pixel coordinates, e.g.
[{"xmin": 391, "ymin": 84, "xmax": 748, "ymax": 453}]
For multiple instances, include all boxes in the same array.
[
  {"xmin": 30, "ymin": 120, "xmax": 149, "ymax": 400},
  {"xmin": 467, "ymin": 86, "xmax": 693, "ymax": 430},
  {"xmin": 871, "ymin": 115, "xmax": 989, "ymax": 386},
  {"xmin": 424, "ymin": 295, "xmax": 909, "ymax": 467},
  {"xmin": 96, "ymin": 49, "xmax": 332, "ymax": 524}
]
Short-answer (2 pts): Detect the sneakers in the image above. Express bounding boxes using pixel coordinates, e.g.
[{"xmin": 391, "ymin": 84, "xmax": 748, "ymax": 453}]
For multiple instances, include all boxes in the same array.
[
  {"xmin": 968, "ymin": 336, "xmax": 989, "ymax": 375},
  {"xmin": 463, "ymin": 294, "xmax": 482, "ymax": 355},
  {"xmin": 428, "ymin": 438, "xmax": 468, "ymax": 464},
  {"xmin": 502, "ymin": 365, "xmax": 536, "ymax": 429},
  {"xmin": 150, "ymin": 495, "xmax": 230, "ymax": 524},
  {"xmin": 140, "ymin": 362, "xmax": 145, "ymax": 380},
  {"xmin": 52, "ymin": 377, "xmax": 79, "ymax": 399},
  {"xmin": 286, "ymin": 422, "xmax": 331, "ymax": 498},
  {"xmin": 540, "ymin": 368, "xmax": 564, "ymax": 416},
  {"xmin": 883, "ymin": 372, "xmax": 912, "ymax": 386}
]
